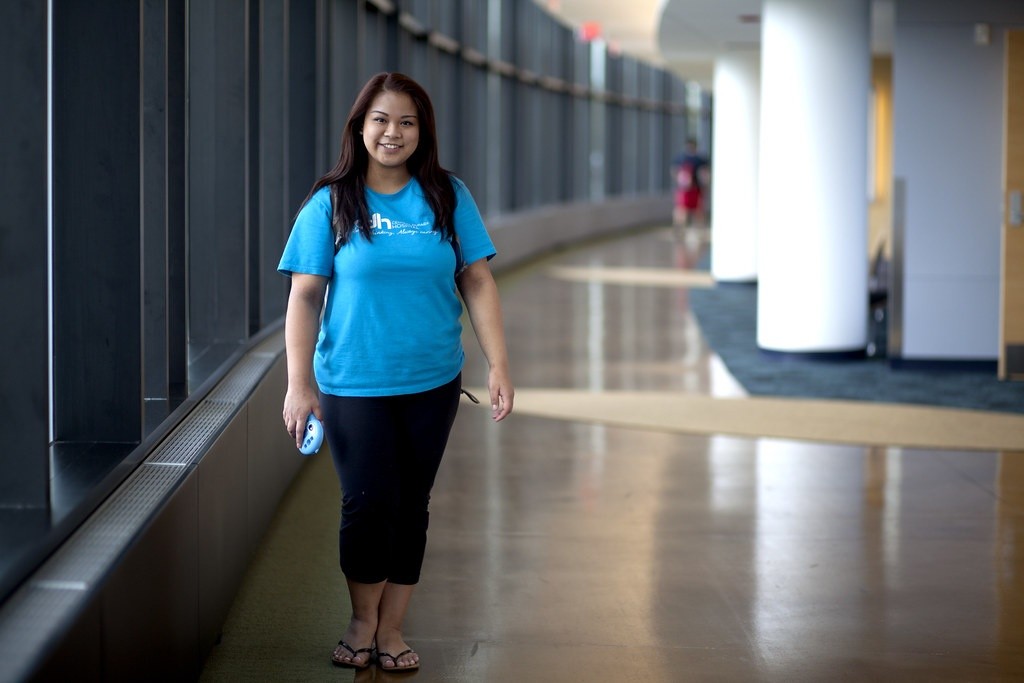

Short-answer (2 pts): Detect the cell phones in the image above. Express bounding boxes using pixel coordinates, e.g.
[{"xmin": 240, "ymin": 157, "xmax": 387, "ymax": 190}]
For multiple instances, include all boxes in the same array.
[{"xmin": 298, "ymin": 411, "xmax": 323, "ymax": 454}]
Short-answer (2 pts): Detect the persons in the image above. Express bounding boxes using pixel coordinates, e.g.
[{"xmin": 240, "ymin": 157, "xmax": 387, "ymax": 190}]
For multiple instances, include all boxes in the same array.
[
  {"xmin": 274, "ymin": 72, "xmax": 515, "ymax": 670},
  {"xmin": 670, "ymin": 140, "xmax": 711, "ymax": 242}
]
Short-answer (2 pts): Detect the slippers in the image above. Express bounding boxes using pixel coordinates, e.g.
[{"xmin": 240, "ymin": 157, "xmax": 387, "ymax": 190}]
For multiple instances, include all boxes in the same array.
[
  {"xmin": 374, "ymin": 634, "xmax": 419, "ymax": 672},
  {"xmin": 331, "ymin": 640, "xmax": 377, "ymax": 669}
]
